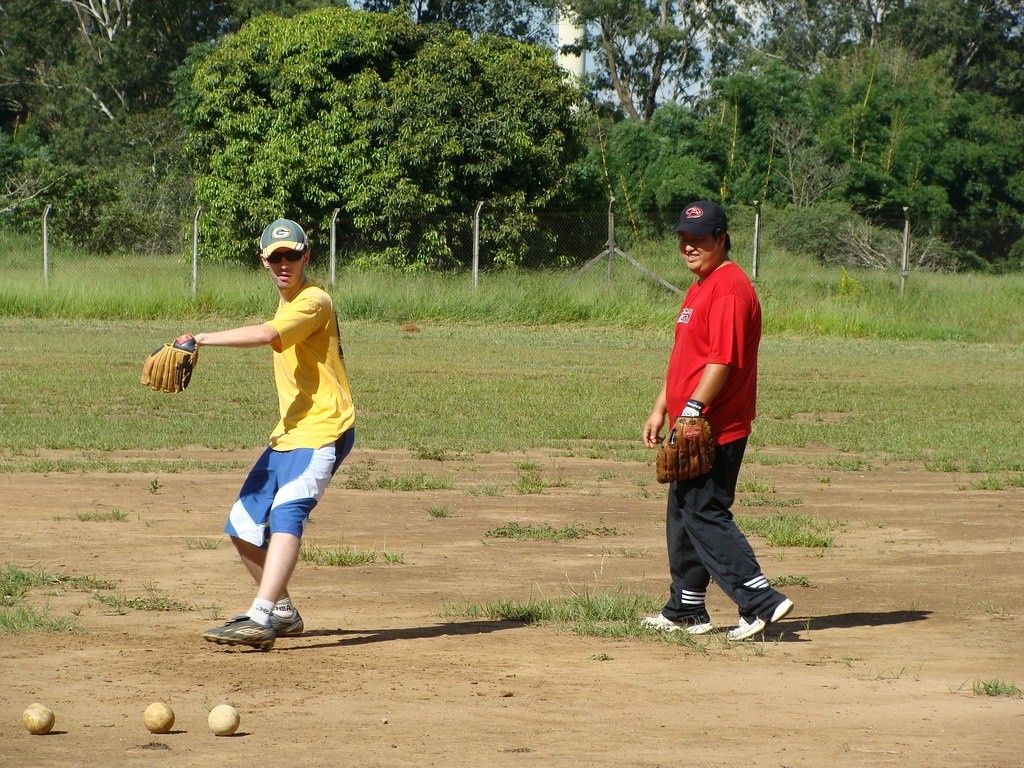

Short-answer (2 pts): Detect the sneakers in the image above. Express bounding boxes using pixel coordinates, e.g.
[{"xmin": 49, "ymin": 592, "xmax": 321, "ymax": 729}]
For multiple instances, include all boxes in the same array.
[
  {"xmin": 727, "ymin": 598, "xmax": 794, "ymax": 641},
  {"xmin": 225, "ymin": 608, "xmax": 304, "ymax": 636},
  {"xmin": 202, "ymin": 615, "xmax": 275, "ymax": 652},
  {"xmin": 641, "ymin": 613, "xmax": 714, "ymax": 635}
]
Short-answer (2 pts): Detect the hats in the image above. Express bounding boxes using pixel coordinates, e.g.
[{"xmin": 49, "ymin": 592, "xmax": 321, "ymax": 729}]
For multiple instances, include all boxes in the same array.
[
  {"xmin": 671, "ymin": 201, "xmax": 727, "ymax": 236},
  {"xmin": 259, "ymin": 218, "xmax": 307, "ymax": 259}
]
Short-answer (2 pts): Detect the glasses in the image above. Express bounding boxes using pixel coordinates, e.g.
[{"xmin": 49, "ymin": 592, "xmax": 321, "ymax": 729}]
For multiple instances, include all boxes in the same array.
[{"xmin": 266, "ymin": 249, "xmax": 304, "ymax": 263}]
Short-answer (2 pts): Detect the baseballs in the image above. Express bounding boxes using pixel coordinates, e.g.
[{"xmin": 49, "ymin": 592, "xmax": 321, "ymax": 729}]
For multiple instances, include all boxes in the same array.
[
  {"xmin": 209, "ymin": 703, "xmax": 241, "ymax": 737},
  {"xmin": 23, "ymin": 702, "xmax": 55, "ymax": 737},
  {"xmin": 144, "ymin": 703, "xmax": 176, "ymax": 734}
]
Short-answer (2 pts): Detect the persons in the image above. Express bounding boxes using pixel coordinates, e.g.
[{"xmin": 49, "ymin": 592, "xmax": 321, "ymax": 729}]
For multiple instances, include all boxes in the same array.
[
  {"xmin": 641, "ymin": 201, "xmax": 795, "ymax": 639},
  {"xmin": 140, "ymin": 217, "xmax": 356, "ymax": 649}
]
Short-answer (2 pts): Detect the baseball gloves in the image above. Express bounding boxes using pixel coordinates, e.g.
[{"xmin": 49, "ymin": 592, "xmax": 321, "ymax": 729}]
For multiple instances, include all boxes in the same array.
[
  {"xmin": 655, "ymin": 415, "xmax": 715, "ymax": 484},
  {"xmin": 140, "ymin": 333, "xmax": 200, "ymax": 395}
]
